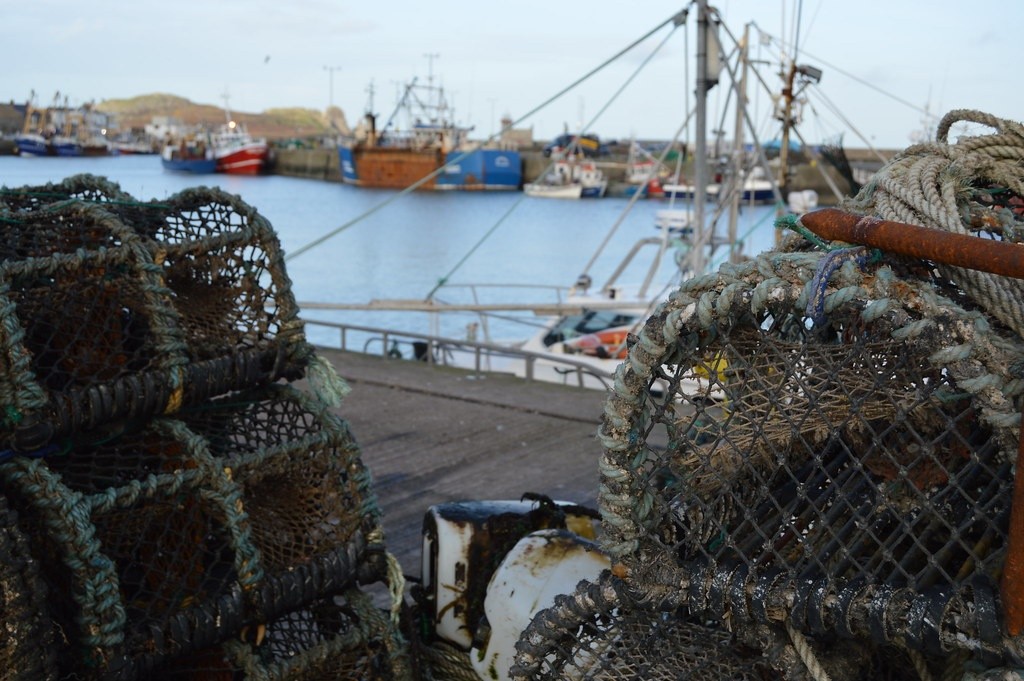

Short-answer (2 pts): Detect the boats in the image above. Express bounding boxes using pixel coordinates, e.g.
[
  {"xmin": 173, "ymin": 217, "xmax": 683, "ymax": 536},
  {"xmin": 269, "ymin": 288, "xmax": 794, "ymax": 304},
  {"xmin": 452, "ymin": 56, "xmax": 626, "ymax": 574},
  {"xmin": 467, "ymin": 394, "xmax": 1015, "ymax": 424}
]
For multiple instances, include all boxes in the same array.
[
  {"xmin": 363, "ymin": 0, "xmax": 818, "ymax": 405},
  {"xmin": 9, "ymin": 84, "xmax": 274, "ymax": 178},
  {"xmin": 521, "ymin": 122, "xmax": 608, "ymax": 201},
  {"xmin": 331, "ymin": 56, "xmax": 523, "ymax": 194},
  {"xmin": 621, "ymin": 20, "xmax": 795, "ymax": 208}
]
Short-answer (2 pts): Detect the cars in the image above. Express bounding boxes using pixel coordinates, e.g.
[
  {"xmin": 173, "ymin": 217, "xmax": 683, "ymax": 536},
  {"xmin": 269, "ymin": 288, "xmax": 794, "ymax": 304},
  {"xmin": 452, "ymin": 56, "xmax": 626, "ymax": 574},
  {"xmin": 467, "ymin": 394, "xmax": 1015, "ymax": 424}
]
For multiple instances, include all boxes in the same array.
[{"xmin": 541, "ymin": 132, "xmax": 602, "ymax": 158}]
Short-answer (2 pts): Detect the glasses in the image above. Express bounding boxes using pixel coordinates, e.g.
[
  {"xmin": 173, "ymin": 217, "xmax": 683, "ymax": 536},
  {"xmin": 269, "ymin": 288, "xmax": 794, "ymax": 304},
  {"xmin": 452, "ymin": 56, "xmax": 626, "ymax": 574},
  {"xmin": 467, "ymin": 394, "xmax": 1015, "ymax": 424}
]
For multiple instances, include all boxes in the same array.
[{"xmin": 577, "ymin": 283, "xmax": 586, "ymax": 286}]
